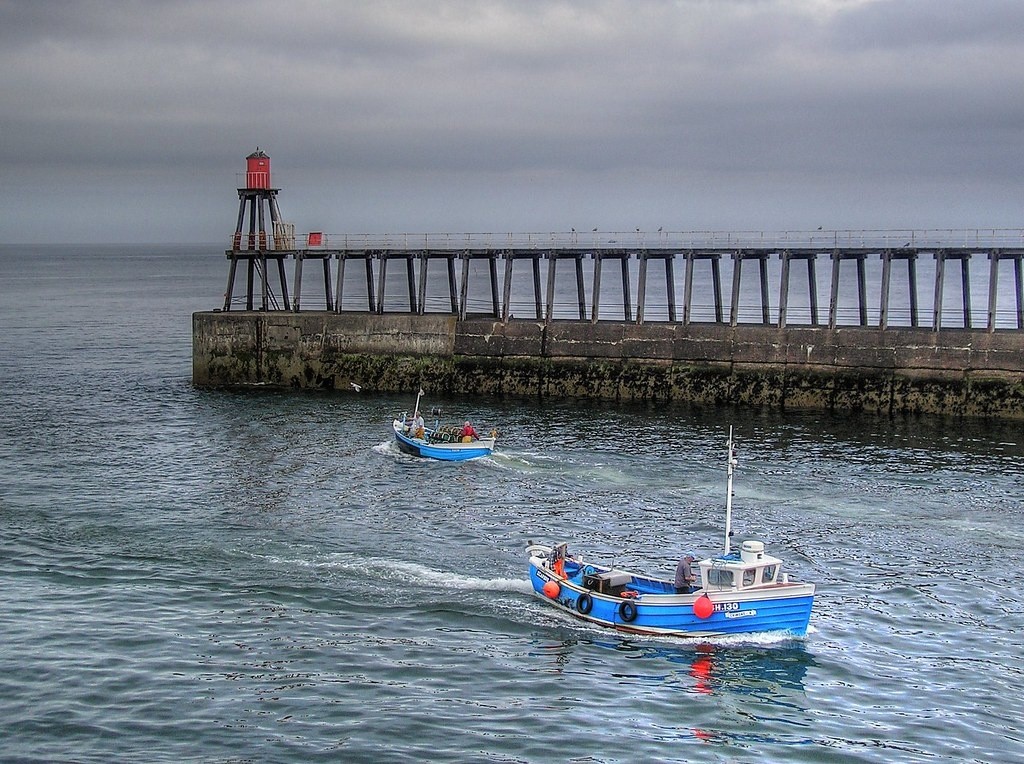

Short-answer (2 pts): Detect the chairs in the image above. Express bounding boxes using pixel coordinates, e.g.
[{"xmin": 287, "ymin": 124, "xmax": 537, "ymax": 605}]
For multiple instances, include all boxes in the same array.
[{"xmin": 427, "ymin": 426, "xmax": 463, "ymax": 444}]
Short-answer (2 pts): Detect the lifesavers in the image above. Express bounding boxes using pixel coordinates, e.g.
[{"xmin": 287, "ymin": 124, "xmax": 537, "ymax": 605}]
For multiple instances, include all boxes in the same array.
[
  {"xmin": 619, "ymin": 600, "xmax": 637, "ymax": 622},
  {"xmin": 576, "ymin": 593, "xmax": 593, "ymax": 614}
]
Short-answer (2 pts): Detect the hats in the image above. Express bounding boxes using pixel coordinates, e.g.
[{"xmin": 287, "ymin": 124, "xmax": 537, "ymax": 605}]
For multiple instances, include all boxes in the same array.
[{"xmin": 686, "ymin": 552, "xmax": 695, "ymax": 558}]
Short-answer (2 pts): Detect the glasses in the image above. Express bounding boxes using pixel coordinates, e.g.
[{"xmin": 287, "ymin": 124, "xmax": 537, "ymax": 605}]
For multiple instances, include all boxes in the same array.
[{"xmin": 691, "ymin": 556, "xmax": 694, "ymax": 560}]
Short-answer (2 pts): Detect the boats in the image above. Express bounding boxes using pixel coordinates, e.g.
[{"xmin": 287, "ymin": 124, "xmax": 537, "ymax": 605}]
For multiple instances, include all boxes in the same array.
[
  {"xmin": 393, "ymin": 387, "xmax": 498, "ymax": 461},
  {"xmin": 525, "ymin": 425, "xmax": 816, "ymax": 647}
]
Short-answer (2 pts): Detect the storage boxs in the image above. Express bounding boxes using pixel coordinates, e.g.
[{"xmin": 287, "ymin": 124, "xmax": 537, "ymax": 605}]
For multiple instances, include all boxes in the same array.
[{"xmin": 583, "ymin": 572, "xmax": 626, "ymax": 597}]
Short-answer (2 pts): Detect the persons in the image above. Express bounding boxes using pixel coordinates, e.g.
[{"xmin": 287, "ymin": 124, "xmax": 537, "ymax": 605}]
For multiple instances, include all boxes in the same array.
[
  {"xmin": 674, "ymin": 552, "xmax": 696, "ymax": 594},
  {"xmin": 415, "ymin": 411, "xmax": 425, "ymax": 439},
  {"xmin": 460, "ymin": 422, "xmax": 479, "ymax": 442}
]
